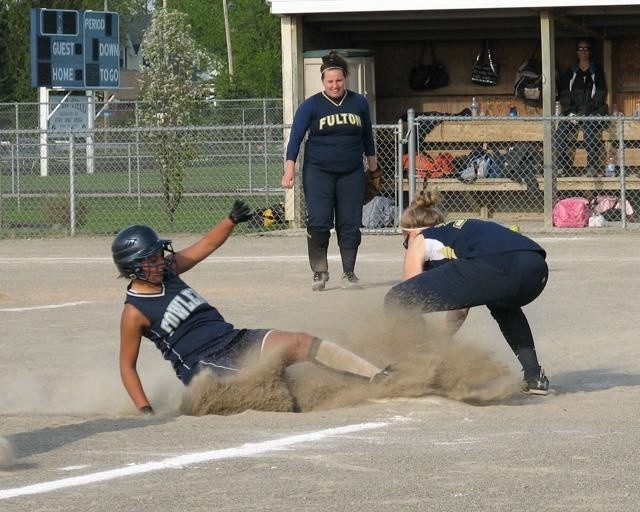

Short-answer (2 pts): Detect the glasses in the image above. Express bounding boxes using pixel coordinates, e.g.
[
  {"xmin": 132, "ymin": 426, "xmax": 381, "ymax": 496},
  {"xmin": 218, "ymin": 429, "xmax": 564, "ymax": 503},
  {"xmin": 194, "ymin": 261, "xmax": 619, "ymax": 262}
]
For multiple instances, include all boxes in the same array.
[
  {"xmin": 403, "ymin": 234, "xmax": 409, "ymax": 249},
  {"xmin": 577, "ymin": 47, "xmax": 590, "ymax": 51}
]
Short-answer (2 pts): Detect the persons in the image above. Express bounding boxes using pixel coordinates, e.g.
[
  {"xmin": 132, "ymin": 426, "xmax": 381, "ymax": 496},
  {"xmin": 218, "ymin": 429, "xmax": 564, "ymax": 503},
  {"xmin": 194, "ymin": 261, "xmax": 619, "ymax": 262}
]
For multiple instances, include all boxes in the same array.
[{"xmin": 553, "ymin": 39, "xmax": 609, "ymax": 177}]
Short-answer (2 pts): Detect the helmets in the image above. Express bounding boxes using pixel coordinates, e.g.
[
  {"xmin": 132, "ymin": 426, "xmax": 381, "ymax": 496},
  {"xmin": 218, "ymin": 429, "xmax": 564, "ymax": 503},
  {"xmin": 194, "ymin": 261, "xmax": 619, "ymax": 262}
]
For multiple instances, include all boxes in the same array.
[{"xmin": 111, "ymin": 224, "xmax": 169, "ymax": 280}]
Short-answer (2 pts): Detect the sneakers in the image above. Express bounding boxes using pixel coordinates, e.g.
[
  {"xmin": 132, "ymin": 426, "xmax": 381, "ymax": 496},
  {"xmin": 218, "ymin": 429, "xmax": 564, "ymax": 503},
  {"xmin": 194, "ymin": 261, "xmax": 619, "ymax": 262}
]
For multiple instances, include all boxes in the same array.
[
  {"xmin": 520, "ymin": 375, "xmax": 549, "ymax": 395},
  {"xmin": 312, "ymin": 271, "xmax": 361, "ymax": 291},
  {"xmin": 366, "ymin": 367, "xmax": 399, "ymax": 385}
]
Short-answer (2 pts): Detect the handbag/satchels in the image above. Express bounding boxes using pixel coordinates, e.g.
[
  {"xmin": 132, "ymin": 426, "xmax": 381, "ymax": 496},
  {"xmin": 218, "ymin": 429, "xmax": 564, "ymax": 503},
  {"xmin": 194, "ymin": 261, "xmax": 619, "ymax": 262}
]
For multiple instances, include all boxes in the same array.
[
  {"xmin": 471, "ymin": 63, "xmax": 498, "ymax": 86},
  {"xmin": 408, "ymin": 62, "xmax": 449, "ymax": 91},
  {"xmin": 514, "ymin": 59, "xmax": 542, "ymax": 107}
]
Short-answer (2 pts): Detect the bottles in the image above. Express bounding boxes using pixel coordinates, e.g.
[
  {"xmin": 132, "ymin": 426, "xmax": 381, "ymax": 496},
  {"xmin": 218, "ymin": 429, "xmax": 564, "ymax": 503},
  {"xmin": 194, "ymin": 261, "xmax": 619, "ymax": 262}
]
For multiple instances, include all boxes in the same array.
[
  {"xmin": 470, "ymin": 96, "xmax": 479, "ymax": 117},
  {"xmin": 634, "ymin": 98, "xmax": 640, "ymax": 117},
  {"xmin": 611, "ymin": 104, "xmax": 619, "ymax": 117},
  {"xmin": 605, "ymin": 157, "xmax": 615, "ymax": 179},
  {"xmin": 555, "ymin": 100, "xmax": 561, "ymax": 117}
]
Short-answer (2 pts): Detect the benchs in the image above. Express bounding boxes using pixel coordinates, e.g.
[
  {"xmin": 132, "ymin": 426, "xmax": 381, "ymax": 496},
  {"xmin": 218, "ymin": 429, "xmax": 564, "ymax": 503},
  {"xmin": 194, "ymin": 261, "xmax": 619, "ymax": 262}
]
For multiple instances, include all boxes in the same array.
[{"xmin": 390, "ymin": 115, "xmax": 639, "ymax": 198}]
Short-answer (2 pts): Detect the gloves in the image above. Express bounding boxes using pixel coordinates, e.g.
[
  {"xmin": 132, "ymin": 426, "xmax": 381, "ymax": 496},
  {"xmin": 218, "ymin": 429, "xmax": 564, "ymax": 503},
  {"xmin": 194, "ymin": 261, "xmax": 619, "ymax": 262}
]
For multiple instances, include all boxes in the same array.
[{"xmin": 229, "ymin": 199, "xmax": 253, "ymax": 224}]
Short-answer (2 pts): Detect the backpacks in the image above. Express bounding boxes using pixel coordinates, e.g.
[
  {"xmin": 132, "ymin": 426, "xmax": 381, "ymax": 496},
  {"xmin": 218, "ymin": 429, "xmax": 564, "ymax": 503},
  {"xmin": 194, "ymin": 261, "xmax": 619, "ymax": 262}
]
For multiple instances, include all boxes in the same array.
[
  {"xmin": 403, "ymin": 148, "xmax": 540, "ymax": 184},
  {"xmin": 553, "ymin": 197, "xmax": 589, "ymax": 228}
]
